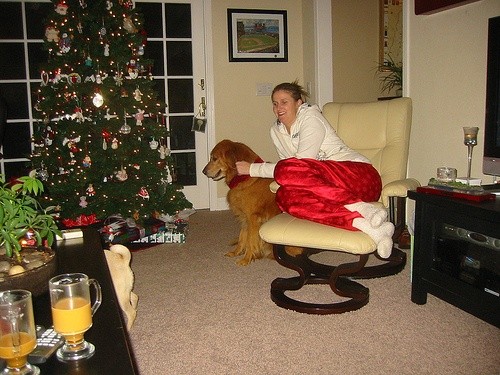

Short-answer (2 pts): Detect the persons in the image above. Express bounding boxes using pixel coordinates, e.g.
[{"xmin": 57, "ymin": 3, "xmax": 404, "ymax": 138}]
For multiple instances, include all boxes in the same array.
[{"xmin": 236, "ymin": 79, "xmax": 395, "ymax": 258}]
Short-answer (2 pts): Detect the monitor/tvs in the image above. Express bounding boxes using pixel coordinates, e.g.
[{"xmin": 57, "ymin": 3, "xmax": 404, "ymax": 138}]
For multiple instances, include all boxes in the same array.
[{"xmin": 482, "ymin": 16, "xmax": 500, "ymax": 195}]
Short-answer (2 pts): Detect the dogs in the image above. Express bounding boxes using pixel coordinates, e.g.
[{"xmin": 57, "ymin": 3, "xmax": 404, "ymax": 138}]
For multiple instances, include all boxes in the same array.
[
  {"xmin": 203, "ymin": 139, "xmax": 303, "ymax": 265},
  {"xmin": 104, "ymin": 244, "xmax": 139, "ymax": 330}
]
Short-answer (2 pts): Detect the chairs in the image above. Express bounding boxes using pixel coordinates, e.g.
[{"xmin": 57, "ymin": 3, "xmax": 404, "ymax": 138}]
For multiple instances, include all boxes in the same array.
[{"xmin": 259, "ymin": 96, "xmax": 422, "ymax": 317}]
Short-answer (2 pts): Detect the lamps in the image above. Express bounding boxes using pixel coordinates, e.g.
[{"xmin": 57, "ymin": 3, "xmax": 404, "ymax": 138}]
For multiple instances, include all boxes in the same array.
[{"xmin": 463, "ymin": 126, "xmax": 479, "ymax": 178}]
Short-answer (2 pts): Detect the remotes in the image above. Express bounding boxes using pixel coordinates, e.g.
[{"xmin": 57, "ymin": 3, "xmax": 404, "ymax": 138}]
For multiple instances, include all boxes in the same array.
[{"xmin": 27, "ymin": 324, "xmax": 65, "ymax": 364}]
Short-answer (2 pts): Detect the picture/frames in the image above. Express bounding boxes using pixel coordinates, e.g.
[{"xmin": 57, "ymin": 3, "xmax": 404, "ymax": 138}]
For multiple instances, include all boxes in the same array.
[{"xmin": 226, "ymin": 8, "xmax": 289, "ymax": 62}]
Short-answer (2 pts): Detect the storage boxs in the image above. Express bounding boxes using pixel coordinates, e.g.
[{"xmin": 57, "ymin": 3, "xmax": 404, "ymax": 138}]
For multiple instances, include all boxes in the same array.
[{"xmin": 96, "ymin": 219, "xmax": 167, "ymax": 245}]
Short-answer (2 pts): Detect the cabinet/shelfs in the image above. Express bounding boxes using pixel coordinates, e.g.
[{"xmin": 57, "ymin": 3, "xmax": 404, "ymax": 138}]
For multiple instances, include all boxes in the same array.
[{"xmin": 405, "ymin": 182, "xmax": 500, "ymax": 331}]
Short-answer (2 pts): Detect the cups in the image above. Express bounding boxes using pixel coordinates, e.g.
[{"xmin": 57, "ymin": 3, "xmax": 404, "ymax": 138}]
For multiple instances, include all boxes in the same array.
[
  {"xmin": 0, "ymin": 289, "xmax": 40, "ymax": 375},
  {"xmin": 48, "ymin": 272, "xmax": 102, "ymax": 362},
  {"xmin": 437, "ymin": 167, "xmax": 458, "ymax": 181}
]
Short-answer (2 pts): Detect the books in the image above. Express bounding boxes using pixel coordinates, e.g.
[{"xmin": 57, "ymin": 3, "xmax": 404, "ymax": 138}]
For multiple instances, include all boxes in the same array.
[
  {"xmin": 56, "ymin": 228, "xmax": 83, "ymax": 240},
  {"xmin": 456, "ymin": 177, "xmax": 482, "ymax": 187},
  {"xmin": 20, "ymin": 238, "xmax": 51, "ymax": 249}
]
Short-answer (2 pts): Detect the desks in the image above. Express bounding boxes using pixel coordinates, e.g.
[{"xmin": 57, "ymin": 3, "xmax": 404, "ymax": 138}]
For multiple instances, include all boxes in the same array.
[{"xmin": 0, "ymin": 223, "xmax": 140, "ymax": 375}]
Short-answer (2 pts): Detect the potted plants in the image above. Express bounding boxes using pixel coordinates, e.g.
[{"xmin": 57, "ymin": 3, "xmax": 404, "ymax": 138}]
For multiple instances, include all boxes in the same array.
[
  {"xmin": 0, "ymin": 168, "xmax": 64, "ymax": 300},
  {"xmin": 371, "ymin": 51, "xmax": 404, "ymax": 97}
]
